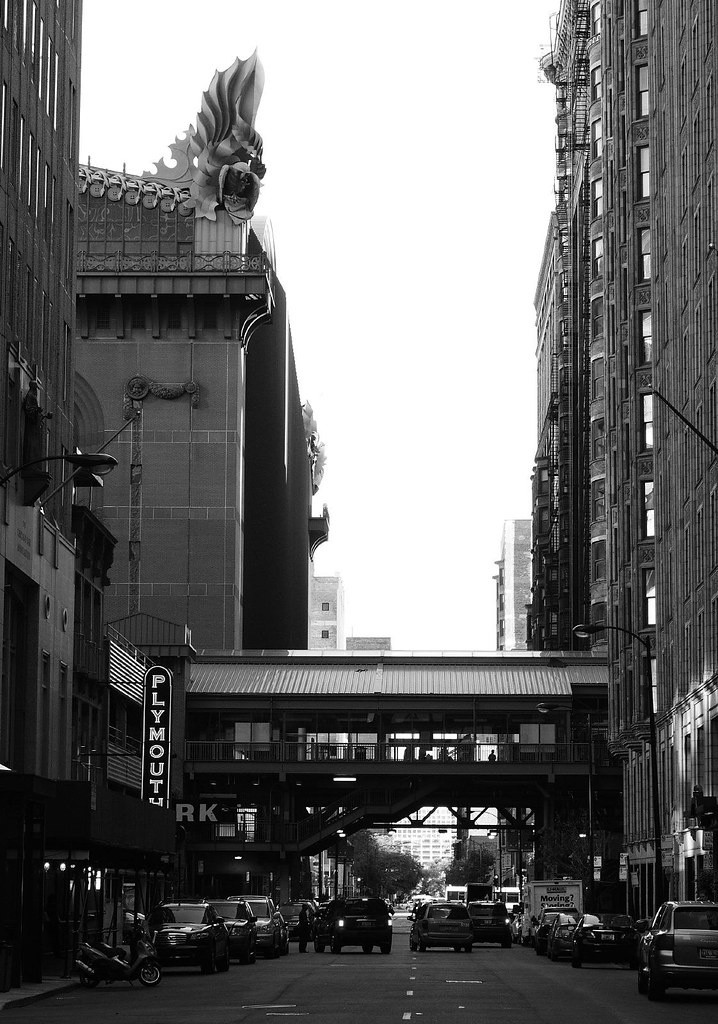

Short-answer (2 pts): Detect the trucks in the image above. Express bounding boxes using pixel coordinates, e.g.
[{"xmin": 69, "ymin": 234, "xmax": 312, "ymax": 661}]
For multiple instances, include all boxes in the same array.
[{"xmin": 512, "ymin": 877, "xmax": 584, "ymax": 948}]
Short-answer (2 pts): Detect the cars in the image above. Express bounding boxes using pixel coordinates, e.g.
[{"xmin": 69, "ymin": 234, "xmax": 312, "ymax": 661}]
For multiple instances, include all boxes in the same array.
[
  {"xmin": 532, "ymin": 904, "xmax": 579, "ymax": 956},
  {"xmin": 547, "ymin": 913, "xmax": 586, "ymax": 963},
  {"xmin": 567, "ymin": 913, "xmax": 638, "ymax": 968},
  {"xmin": 507, "ymin": 910, "xmax": 521, "ymax": 944}
]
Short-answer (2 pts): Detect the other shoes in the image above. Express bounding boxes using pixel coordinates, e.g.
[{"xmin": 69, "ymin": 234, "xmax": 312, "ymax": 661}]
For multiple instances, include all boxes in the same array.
[{"xmin": 300, "ymin": 950, "xmax": 308, "ymax": 953}]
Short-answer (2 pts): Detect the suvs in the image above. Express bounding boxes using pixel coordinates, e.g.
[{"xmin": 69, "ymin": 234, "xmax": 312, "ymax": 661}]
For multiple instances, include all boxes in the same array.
[
  {"xmin": 225, "ymin": 894, "xmax": 330, "ymax": 960},
  {"xmin": 198, "ymin": 896, "xmax": 258, "ymax": 964},
  {"xmin": 314, "ymin": 895, "xmax": 394, "ymax": 953},
  {"xmin": 139, "ymin": 899, "xmax": 230, "ymax": 974},
  {"xmin": 633, "ymin": 899, "xmax": 718, "ymax": 1002},
  {"xmin": 462, "ymin": 898, "xmax": 513, "ymax": 949},
  {"xmin": 407, "ymin": 900, "xmax": 475, "ymax": 953}
]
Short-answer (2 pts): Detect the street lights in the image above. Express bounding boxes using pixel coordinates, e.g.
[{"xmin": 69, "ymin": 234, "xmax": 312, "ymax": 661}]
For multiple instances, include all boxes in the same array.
[
  {"xmin": 464, "ymin": 809, "xmax": 502, "ymax": 901},
  {"xmin": 537, "ymin": 702, "xmax": 597, "ymax": 915},
  {"xmin": 572, "ymin": 623, "xmax": 665, "ymax": 916}
]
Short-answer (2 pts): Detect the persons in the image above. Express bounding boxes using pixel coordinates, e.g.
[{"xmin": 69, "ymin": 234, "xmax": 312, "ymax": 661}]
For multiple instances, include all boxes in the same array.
[
  {"xmin": 488, "ymin": 750, "xmax": 496, "ymax": 761},
  {"xmin": 298, "ymin": 904, "xmax": 311, "ymax": 953}
]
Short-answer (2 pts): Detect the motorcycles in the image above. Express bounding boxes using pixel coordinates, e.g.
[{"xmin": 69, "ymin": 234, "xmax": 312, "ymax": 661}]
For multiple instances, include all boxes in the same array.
[{"xmin": 72, "ymin": 917, "xmax": 162, "ymax": 988}]
